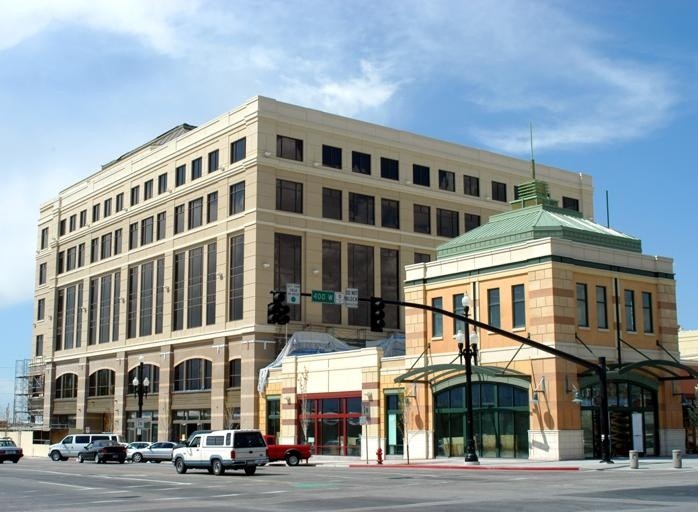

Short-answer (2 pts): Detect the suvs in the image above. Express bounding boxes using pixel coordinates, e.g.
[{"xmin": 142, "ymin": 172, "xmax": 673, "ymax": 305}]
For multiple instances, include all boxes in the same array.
[{"xmin": 171, "ymin": 429, "xmax": 270, "ymax": 475}]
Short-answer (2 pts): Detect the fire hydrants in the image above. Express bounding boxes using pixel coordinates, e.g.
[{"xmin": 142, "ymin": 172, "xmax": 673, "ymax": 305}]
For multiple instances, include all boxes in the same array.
[{"xmin": 376, "ymin": 448, "xmax": 382, "ymax": 464}]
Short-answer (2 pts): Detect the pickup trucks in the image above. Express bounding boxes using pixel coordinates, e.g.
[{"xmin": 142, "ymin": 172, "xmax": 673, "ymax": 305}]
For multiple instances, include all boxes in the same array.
[{"xmin": 263, "ymin": 434, "xmax": 313, "ymax": 466}]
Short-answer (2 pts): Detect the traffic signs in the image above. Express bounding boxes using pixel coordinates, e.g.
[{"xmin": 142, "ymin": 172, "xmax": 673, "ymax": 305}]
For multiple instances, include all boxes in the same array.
[{"xmin": 287, "ymin": 284, "xmax": 358, "ymax": 309}]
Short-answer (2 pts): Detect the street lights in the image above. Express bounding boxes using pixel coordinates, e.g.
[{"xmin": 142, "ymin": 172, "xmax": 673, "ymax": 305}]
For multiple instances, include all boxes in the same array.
[
  {"xmin": 132, "ymin": 353, "xmax": 149, "ymax": 442},
  {"xmin": 456, "ymin": 291, "xmax": 479, "ymax": 466}
]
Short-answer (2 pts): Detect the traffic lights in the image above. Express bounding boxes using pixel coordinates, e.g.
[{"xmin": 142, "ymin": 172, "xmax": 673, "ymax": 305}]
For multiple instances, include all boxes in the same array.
[
  {"xmin": 267, "ymin": 292, "xmax": 290, "ymax": 325},
  {"xmin": 371, "ymin": 296, "xmax": 385, "ymax": 332}
]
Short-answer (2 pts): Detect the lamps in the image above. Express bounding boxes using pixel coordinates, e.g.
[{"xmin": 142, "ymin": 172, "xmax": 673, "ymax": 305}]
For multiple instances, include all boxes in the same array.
[
  {"xmin": 402, "ymin": 382, "xmax": 416, "ymax": 406},
  {"xmin": 565, "ymin": 376, "xmax": 583, "ymax": 405},
  {"xmin": 528, "ymin": 376, "xmax": 544, "ymax": 404}
]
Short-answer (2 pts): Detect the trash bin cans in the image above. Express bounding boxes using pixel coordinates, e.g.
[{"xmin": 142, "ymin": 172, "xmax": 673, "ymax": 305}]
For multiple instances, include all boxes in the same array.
[
  {"xmin": 672, "ymin": 449, "xmax": 682, "ymax": 468},
  {"xmin": 629, "ymin": 450, "xmax": 639, "ymax": 469}
]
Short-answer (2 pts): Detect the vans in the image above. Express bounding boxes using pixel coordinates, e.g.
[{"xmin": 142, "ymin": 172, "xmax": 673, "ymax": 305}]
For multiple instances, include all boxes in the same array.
[{"xmin": 48, "ymin": 433, "xmax": 120, "ymax": 461}]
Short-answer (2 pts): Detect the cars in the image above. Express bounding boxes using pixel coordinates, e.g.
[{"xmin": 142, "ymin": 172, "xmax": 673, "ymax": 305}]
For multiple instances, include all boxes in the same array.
[
  {"xmin": 0, "ymin": 437, "xmax": 24, "ymax": 463},
  {"xmin": 78, "ymin": 440, "xmax": 178, "ymax": 464}
]
